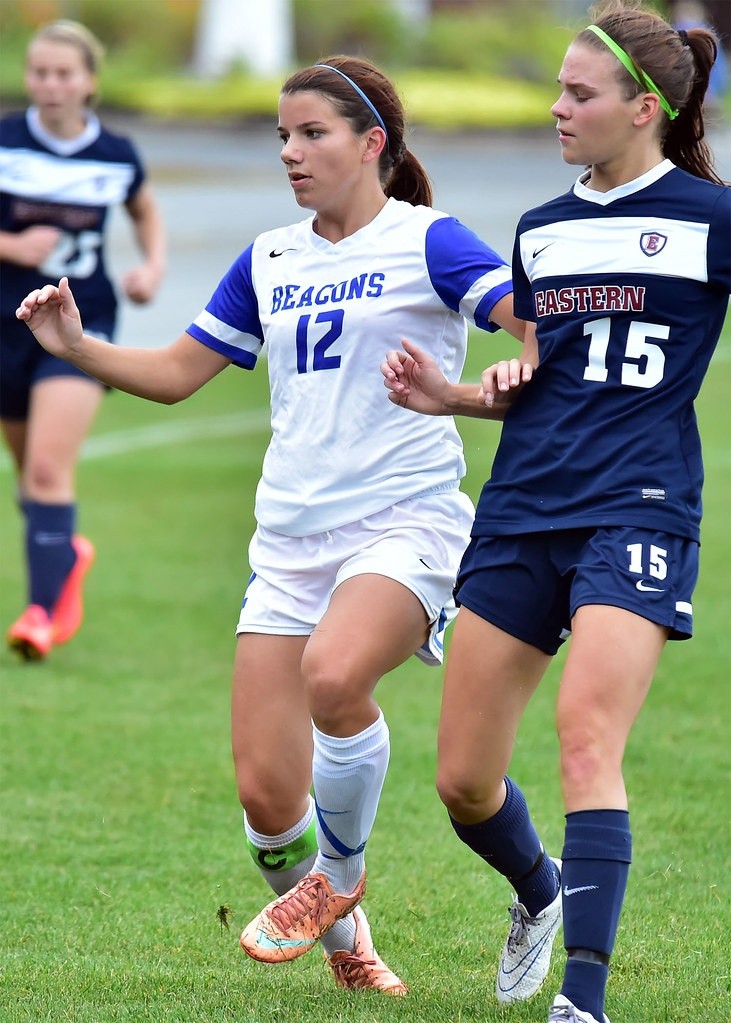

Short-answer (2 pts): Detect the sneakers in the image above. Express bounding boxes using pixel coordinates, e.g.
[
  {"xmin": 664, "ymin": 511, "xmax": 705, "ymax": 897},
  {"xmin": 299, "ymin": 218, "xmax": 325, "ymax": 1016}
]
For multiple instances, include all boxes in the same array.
[
  {"xmin": 8, "ymin": 534, "xmax": 95, "ymax": 662},
  {"xmin": 240, "ymin": 870, "xmax": 368, "ymax": 964},
  {"xmin": 493, "ymin": 857, "xmax": 611, "ymax": 1023},
  {"xmin": 322, "ymin": 900, "xmax": 410, "ymax": 999}
]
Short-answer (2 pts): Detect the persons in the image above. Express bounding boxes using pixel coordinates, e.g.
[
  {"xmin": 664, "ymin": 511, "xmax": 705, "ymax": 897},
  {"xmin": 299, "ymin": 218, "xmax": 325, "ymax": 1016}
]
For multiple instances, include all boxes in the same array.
[
  {"xmin": 13, "ymin": 58, "xmax": 539, "ymax": 1000},
  {"xmin": 380, "ymin": 2, "xmax": 731, "ymax": 1022},
  {"xmin": 0, "ymin": 19, "xmax": 167, "ymax": 661}
]
